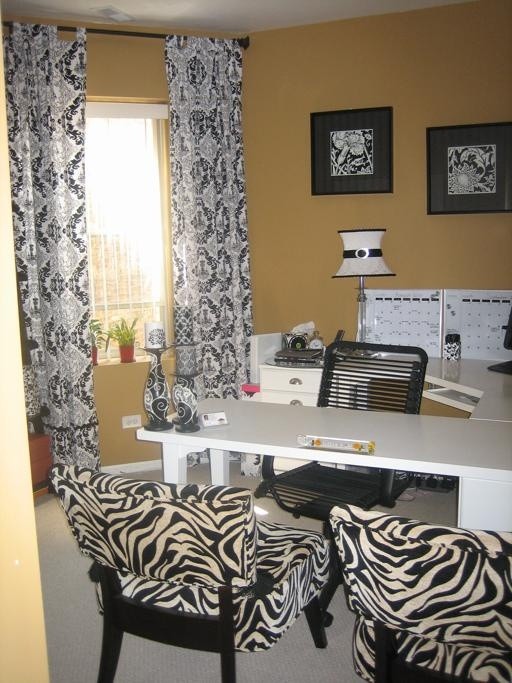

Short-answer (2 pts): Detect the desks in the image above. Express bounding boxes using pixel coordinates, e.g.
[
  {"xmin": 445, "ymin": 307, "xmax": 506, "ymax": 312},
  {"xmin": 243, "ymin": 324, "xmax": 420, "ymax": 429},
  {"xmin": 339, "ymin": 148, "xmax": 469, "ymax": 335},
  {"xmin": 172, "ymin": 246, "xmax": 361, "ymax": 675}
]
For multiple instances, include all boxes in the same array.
[
  {"xmin": 134, "ymin": 398, "xmax": 510, "ymax": 545},
  {"xmin": 375, "ymin": 352, "xmax": 509, "ymax": 421}
]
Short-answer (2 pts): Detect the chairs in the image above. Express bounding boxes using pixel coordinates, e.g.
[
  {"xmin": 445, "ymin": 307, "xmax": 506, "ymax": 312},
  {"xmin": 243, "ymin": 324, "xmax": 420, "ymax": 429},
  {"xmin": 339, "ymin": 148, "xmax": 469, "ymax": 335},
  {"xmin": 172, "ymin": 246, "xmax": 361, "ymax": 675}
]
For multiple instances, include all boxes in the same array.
[
  {"xmin": 49, "ymin": 463, "xmax": 331, "ymax": 681},
  {"xmin": 328, "ymin": 503, "xmax": 510, "ymax": 682},
  {"xmin": 253, "ymin": 340, "xmax": 429, "ymax": 540}
]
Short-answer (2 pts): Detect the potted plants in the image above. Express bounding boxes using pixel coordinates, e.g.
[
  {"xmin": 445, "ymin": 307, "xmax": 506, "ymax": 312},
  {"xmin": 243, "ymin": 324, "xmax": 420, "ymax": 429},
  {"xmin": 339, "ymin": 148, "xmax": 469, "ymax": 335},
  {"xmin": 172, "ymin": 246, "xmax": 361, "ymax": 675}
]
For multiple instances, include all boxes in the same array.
[{"xmin": 88, "ymin": 319, "xmax": 138, "ymax": 366}]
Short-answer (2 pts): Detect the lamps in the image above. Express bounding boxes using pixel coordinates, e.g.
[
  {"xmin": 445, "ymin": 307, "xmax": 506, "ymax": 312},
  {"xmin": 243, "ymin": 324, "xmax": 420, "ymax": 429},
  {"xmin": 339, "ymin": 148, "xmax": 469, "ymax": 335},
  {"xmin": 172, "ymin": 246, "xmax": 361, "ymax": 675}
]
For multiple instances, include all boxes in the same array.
[{"xmin": 331, "ymin": 227, "xmax": 397, "ymax": 356}]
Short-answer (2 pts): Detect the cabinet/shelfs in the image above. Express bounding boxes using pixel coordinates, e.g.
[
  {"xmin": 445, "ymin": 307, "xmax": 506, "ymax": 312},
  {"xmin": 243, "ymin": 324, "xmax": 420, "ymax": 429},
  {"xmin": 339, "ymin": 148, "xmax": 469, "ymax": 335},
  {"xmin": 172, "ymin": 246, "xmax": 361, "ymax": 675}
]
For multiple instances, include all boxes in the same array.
[{"xmin": 259, "ymin": 367, "xmax": 336, "ymax": 475}]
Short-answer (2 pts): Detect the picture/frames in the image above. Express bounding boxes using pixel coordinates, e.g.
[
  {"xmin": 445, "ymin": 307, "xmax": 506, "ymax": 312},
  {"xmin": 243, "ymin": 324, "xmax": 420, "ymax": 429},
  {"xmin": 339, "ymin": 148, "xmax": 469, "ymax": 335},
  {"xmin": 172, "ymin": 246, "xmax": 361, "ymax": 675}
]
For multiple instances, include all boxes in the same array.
[{"xmin": 308, "ymin": 104, "xmax": 511, "ymax": 215}]
